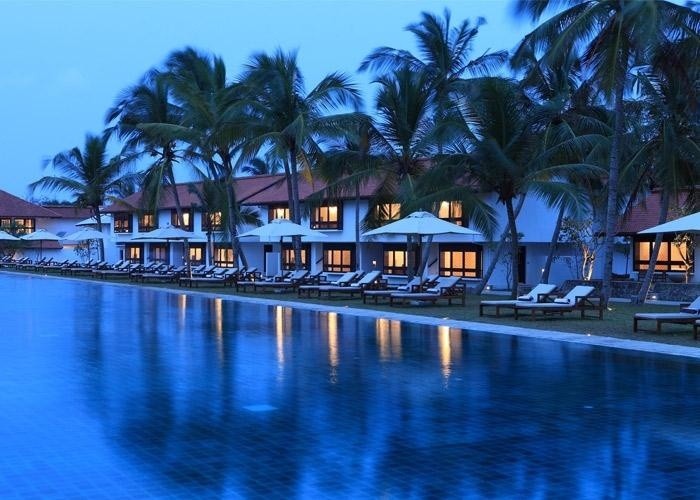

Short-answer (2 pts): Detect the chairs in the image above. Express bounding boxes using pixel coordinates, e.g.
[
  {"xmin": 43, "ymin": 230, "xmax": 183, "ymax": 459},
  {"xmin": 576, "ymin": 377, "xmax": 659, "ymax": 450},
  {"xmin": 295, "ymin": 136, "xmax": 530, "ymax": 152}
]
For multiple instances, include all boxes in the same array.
[
  {"xmin": 478, "ymin": 283, "xmax": 562, "ymax": 319},
  {"xmin": 513, "ymin": 285, "xmax": 605, "ymax": 322},
  {"xmin": 236, "ymin": 269, "xmax": 465, "ymax": 305},
  {"xmin": 692, "ymin": 319, "xmax": 700, "ymax": 340},
  {"xmin": 633, "ymin": 295, "xmax": 700, "ymax": 335},
  {"xmin": 1, "ymin": 255, "xmax": 262, "ymax": 287}
]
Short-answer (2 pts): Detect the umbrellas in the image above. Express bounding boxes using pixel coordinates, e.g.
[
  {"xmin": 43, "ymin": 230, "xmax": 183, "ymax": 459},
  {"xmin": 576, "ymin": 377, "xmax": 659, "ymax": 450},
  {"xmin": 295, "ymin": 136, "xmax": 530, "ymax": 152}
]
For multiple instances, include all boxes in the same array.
[
  {"xmin": 130, "ymin": 220, "xmax": 206, "ymax": 275},
  {"xmin": 635, "ymin": 210, "xmax": 700, "ymax": 236},
  {"xmin": 360, "ymin": 207, "xmax": 482, "ymax": 295},
  {"xmin": 234, "ymin": 214, "xmax": 327, "ymax": 283},
  {"xmin": 0, "ymin": 209, "xmax": 105, "ymax": 277}
]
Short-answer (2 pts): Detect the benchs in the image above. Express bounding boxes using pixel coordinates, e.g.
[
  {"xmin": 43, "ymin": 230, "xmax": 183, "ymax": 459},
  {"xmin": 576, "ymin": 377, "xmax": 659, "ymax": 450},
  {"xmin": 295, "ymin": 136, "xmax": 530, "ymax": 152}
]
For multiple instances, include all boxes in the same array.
[{"xmin": 637, "ymin": 269, "xmax": 669, "ymax": 282}]
